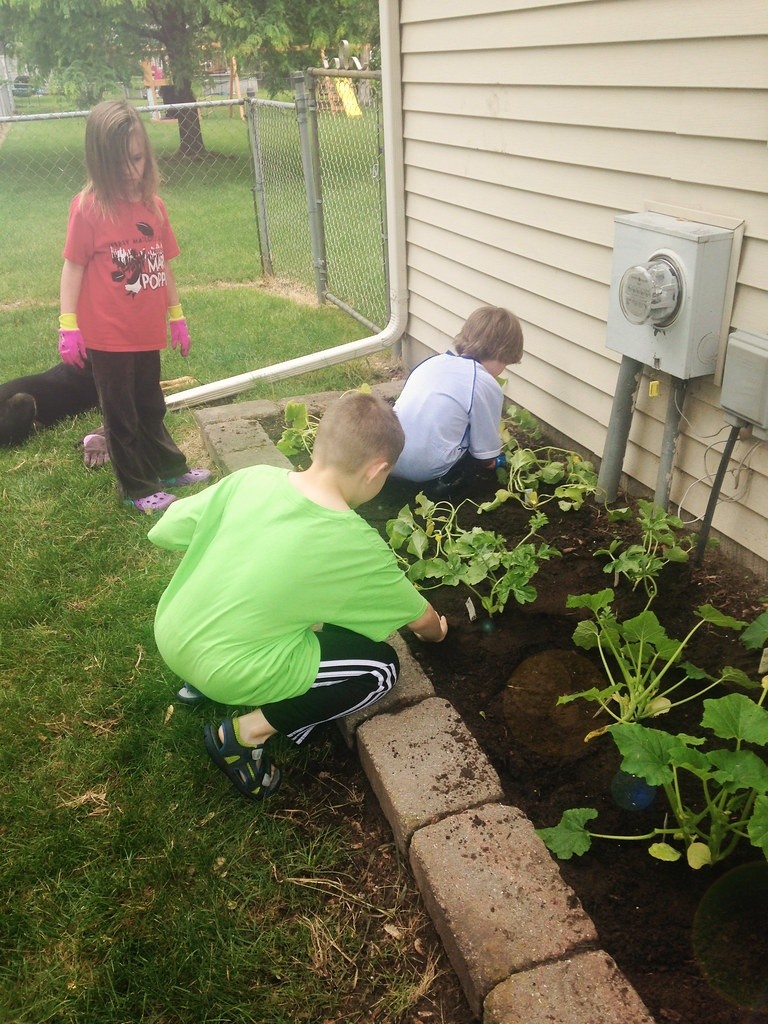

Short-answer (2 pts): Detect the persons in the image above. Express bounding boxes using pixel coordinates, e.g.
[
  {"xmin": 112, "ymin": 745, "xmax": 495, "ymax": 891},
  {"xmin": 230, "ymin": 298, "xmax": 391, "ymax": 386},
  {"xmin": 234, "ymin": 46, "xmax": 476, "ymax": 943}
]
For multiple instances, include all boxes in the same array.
[
  {"xmin": 145, "ymin": 391, "xmax": 449, "ymax": 801},
  {"xmin": 388, "ymin": 306, "xmax": 525, "ymax": 504},
  {"xmin": 58, "ymin": 98, "xmax": 212, "ymax": 514}
]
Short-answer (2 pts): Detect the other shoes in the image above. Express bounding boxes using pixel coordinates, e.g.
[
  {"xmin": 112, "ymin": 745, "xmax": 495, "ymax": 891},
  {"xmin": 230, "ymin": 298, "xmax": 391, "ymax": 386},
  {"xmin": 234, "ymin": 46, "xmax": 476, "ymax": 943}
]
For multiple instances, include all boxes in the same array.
[{"xmin": 425, "ymin": 469, "xmax": 463, "ymax": 500}]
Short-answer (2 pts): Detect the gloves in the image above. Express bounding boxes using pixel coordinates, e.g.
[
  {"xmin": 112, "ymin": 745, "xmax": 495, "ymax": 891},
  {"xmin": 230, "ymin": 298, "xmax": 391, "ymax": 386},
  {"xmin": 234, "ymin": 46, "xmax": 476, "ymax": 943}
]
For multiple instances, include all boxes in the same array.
[
  {"xmin": 83, "ymin": 434, "xmax": 111, "ymax": 468},
  {"xmin": 168, "ymin": 303, "xmax": 191, "ymax": 357},
  {"xmin": 493, "ymin": 454, "xmax": 508, "ymax": 469},
  {"xmin": 57, "ymin": 313, "xmax": 86, "ymax": 369}
]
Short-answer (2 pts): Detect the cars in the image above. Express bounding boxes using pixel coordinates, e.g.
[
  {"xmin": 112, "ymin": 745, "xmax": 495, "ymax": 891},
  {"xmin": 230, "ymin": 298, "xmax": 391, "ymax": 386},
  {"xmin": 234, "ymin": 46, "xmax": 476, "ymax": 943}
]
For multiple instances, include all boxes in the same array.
[{"xmin": 11, "ymin": 75, "xmax": 34, "ymax": 98}]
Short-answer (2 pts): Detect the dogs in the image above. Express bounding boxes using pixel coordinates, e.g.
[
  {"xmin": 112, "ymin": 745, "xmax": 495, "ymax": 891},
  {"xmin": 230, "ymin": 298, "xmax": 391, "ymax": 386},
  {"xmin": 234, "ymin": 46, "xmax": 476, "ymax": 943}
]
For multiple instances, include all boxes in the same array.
[{"xmin": 0, "ymin": 361, "xmax": 198, "ymax": 450}]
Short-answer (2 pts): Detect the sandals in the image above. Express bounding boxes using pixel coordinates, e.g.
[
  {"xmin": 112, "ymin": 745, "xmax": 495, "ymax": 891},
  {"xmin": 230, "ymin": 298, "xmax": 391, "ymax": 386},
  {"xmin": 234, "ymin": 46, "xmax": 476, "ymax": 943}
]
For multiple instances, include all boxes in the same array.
[
  {"xmin": 203, "ymin": 717, "xmax": 283, "ymax": 800},
  {"xmin": 178, "ymin": 681, "xmax": 208, "ymax": 704},
  {"xmin": 160, "ymin": 464, "xmax": 212, "ymax": 484},
  {"xmin": 122, "ymin": 491, "xmax": 176, "ymax": 513}
]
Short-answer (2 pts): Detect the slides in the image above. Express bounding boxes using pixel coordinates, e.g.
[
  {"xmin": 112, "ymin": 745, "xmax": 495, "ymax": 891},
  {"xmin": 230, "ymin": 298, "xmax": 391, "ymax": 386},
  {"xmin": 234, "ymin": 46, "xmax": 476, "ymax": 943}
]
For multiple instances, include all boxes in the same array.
[{"xmin": 334, "ymin": 77, "xmax": 363, "ymax": 117}]
